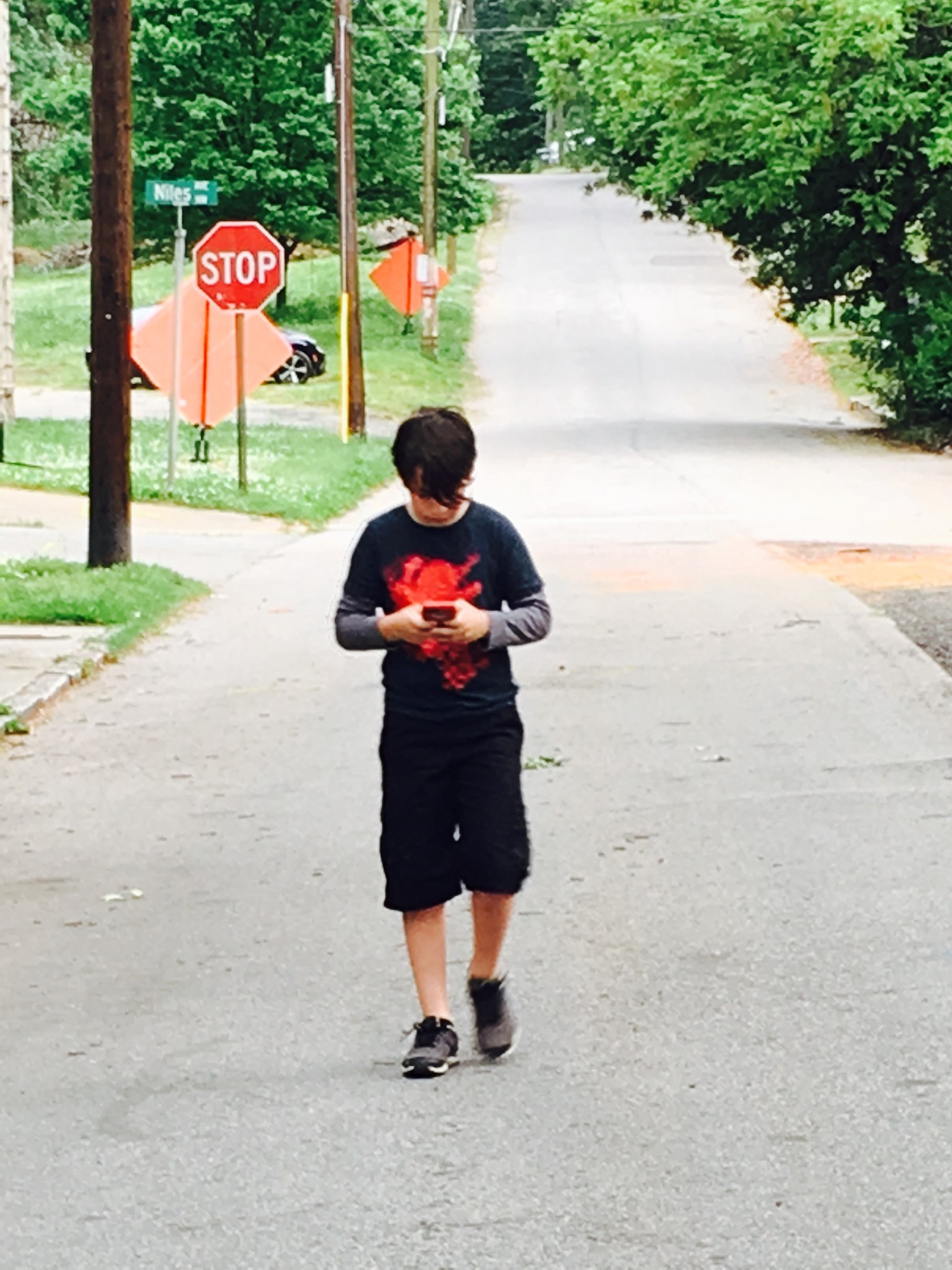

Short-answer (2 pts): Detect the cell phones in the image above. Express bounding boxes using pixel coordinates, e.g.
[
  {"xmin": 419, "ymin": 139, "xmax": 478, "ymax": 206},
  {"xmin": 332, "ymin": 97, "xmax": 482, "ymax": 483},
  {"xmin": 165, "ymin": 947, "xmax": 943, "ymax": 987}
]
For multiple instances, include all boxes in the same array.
[{"xmin": 423, "ymin": 605, "xmax": 456, "ymax": 629}]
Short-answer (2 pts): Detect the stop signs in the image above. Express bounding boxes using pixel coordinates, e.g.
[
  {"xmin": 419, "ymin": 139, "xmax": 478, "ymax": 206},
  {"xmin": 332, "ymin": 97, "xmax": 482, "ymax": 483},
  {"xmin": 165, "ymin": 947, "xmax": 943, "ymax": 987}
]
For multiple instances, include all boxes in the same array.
[{"xmin": 192, "ymin": 223, "xmax": 284, "ymax": 310}]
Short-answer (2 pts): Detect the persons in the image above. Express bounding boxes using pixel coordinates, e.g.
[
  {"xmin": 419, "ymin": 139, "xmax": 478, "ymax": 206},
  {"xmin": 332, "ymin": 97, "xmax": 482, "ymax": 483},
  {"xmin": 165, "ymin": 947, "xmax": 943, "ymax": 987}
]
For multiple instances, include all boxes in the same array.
[{"xmin": 332, "ymin": 404, "xmax": 552, "ymax": 1078}]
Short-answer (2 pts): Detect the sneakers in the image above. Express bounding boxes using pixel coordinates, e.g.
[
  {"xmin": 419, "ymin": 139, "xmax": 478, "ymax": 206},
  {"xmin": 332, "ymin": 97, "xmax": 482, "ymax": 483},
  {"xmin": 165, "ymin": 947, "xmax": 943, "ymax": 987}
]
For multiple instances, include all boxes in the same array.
[
  {"xmin": 467, "ymin": 971, "xmax": 512, "ymax": 1057},
  {"xmin": 402, "ymin": 1016, "xmax": 459, "ymax": 1078}
]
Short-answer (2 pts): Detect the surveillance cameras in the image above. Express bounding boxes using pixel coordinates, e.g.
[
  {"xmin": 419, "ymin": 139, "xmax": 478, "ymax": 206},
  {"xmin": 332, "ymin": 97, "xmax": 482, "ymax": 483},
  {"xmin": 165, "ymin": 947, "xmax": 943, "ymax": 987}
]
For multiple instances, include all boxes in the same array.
[
  {"xmin": 438, "ymin": 94, "xmax": 446, "ymax": 125},
  {"xmin": 325, "ymin": 63, "xmax": 336, "ymax": 104}
]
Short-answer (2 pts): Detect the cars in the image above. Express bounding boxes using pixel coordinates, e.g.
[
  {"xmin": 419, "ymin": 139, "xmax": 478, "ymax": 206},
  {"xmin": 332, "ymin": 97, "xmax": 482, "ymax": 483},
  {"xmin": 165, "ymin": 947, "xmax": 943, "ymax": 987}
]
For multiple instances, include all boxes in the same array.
[{"xmin": 85, "ymin": 307, "xmax": 328, "ymax": 386}]
